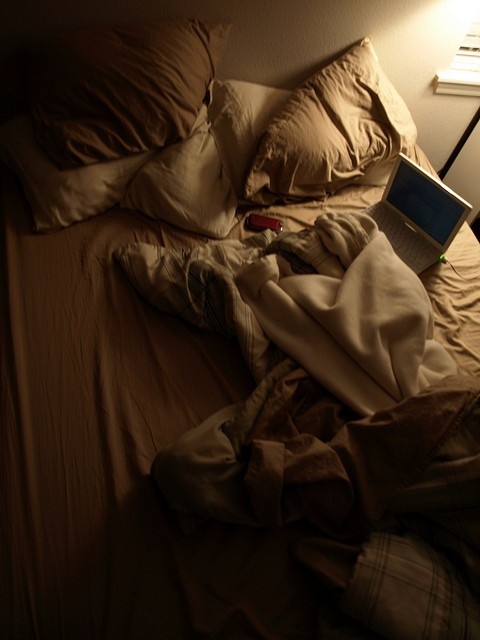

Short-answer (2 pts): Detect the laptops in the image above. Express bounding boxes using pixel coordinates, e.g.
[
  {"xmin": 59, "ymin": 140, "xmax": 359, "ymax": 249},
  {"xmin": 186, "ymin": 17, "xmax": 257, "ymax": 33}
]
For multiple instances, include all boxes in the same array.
[{"xmin": 358, "ymin": 151, "xmax": 474, "ymax": 276}]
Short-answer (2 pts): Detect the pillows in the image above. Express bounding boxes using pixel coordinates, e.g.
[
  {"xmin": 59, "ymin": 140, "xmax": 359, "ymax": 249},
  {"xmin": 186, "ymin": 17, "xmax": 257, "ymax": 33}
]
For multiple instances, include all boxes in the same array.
[
  {"xmin": 242, "ymin": 35, "xmax": 419, "ymax": 203},
  {"xmin": 27, "ymin": 15, "xmax": 215, "ymax": 170},
  {"xmin": 119, "ymin": 76, "xmax": 296, "ymax": 242},
  {"xmin": 11, "ymin": 125, "xmax": 156, "ymax": 230}
]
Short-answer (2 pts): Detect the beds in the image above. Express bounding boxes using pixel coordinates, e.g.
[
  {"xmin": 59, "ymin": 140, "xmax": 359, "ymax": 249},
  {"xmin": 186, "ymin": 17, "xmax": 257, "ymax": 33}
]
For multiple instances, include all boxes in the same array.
[{"xmin": 0, "ymin": 133, "xmax": 480, "ymax": 640}]
